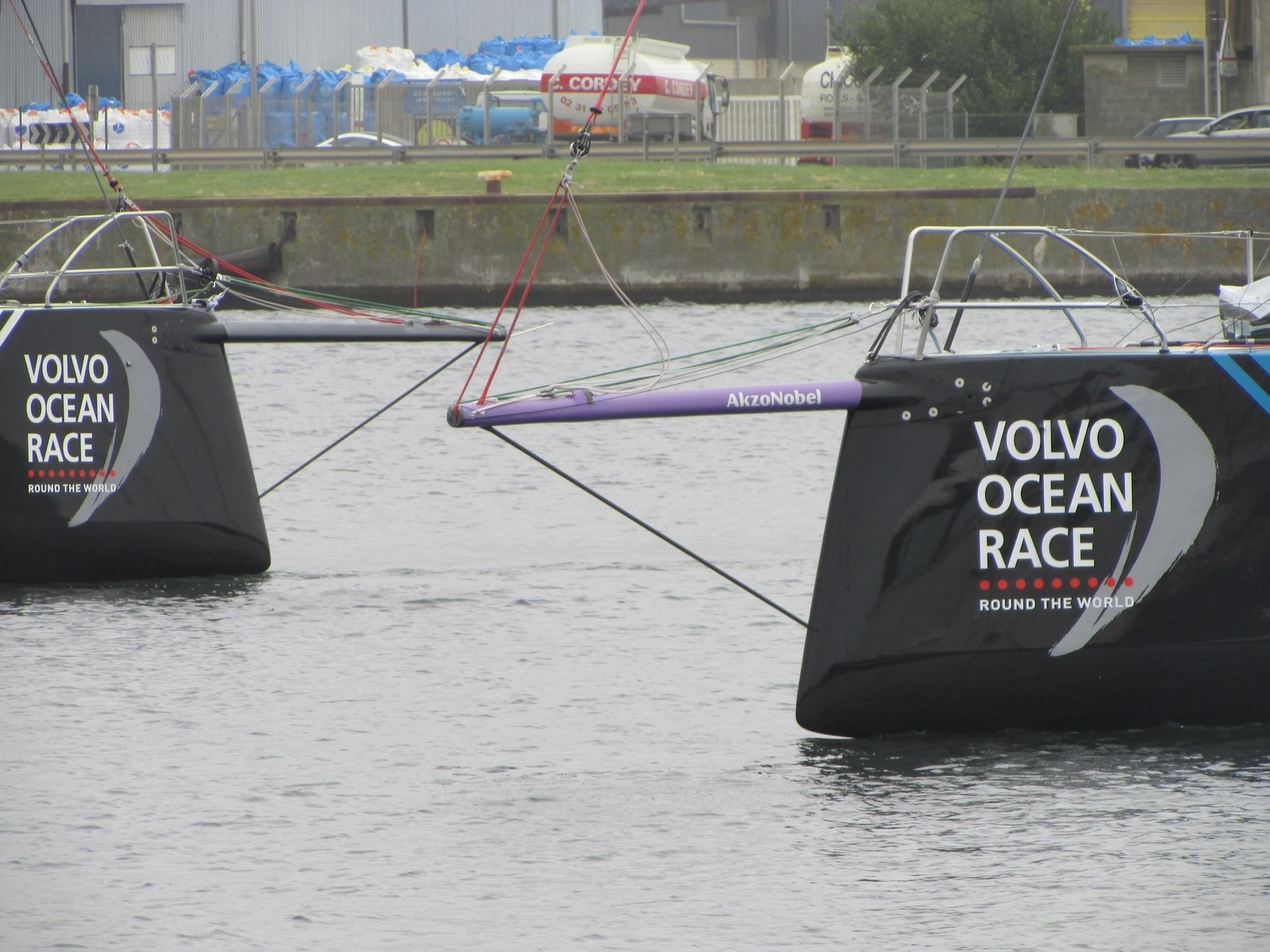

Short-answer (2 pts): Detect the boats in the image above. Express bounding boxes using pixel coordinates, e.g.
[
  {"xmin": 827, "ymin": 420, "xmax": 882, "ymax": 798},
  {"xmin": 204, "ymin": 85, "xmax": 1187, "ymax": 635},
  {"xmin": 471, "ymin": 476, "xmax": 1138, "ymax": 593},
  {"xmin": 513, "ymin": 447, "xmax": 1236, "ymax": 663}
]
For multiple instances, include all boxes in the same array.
[
  {"xmin": 1, "ymin": 2, "xmax": 511, "ymax": 613},
  {"xmin": 436, "ymin": 0, "xmax": 1270, "ymax": 755}
]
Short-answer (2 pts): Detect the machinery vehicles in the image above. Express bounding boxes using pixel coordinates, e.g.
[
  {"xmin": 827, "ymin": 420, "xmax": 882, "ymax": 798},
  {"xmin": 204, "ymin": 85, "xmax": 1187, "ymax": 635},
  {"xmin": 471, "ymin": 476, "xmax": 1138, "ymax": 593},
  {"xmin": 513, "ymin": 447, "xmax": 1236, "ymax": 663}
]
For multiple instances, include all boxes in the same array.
[
  {"xmin": 798, "ymin": 45, "xmax": 888, "ymax": 167},
  {"xmin": 539, "ymin": 34, "xmax": 730, "ymax": 144}
]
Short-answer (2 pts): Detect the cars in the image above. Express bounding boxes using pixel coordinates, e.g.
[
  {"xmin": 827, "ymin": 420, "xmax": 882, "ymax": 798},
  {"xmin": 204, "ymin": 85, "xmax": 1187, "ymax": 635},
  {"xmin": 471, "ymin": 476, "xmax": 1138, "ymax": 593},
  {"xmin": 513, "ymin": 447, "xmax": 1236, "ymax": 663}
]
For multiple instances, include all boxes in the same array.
[
  {"xmin": 1124, "ymin": 115, "xmax": 1220, "ymax": 170},
  {"xmin": 1137, "ymin": 104, "xmax": 1269, "ymax": 171},
  {"xmin": 314, "ymin": 130, "xmax": 415, "ymax": 149}
]
